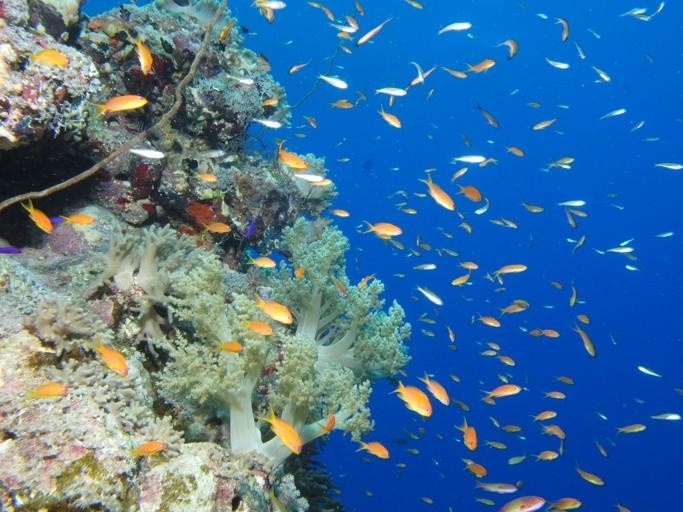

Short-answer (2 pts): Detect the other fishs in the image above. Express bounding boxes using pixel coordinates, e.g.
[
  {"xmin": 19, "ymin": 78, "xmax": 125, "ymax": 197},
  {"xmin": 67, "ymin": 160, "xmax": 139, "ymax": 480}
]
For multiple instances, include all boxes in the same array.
[{"xmin": 155, "ymin": 1, "xmax": 683, "ymax": 512}]
